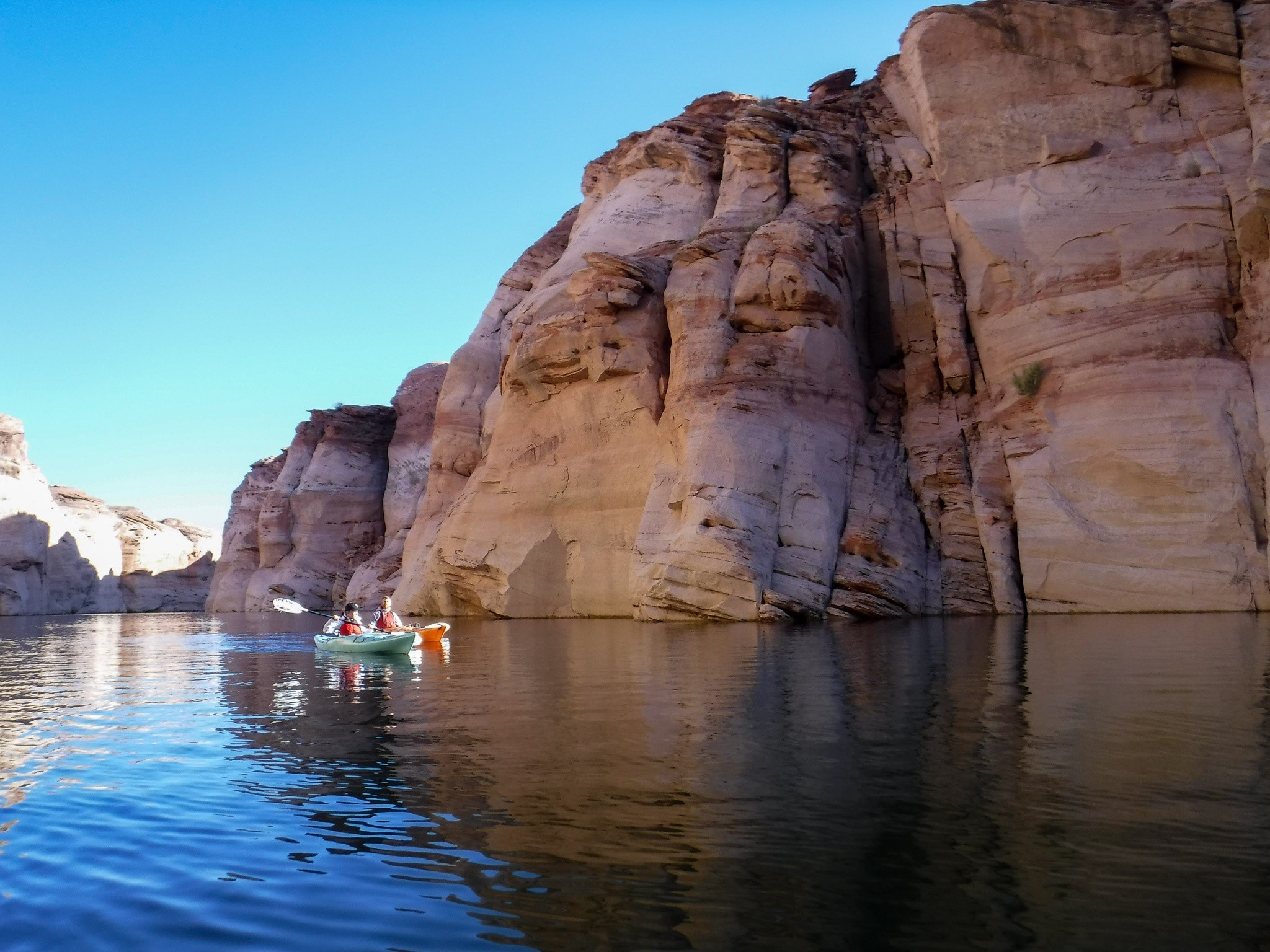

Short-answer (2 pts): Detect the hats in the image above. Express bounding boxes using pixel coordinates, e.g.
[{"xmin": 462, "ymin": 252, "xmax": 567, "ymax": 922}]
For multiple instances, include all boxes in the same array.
[{"xmin": 345, "ymin": 603, "xmax": 361, "ymax": 611}]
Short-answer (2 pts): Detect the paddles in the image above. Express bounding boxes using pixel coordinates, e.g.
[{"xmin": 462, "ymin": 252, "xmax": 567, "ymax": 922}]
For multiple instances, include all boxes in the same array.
[{"xmin": 273, "ymin": 598, "xmax": 423, "ymax": 646}]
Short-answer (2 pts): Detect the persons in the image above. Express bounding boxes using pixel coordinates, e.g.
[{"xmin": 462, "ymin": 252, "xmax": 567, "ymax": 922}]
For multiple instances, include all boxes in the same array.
[
  {"xmin": 368, "ymin": 595, "xmax": 404, "ymax": 629},
  {"xmin": 323, "ymin": 602, "xmax": 374, "ymax": 637}
]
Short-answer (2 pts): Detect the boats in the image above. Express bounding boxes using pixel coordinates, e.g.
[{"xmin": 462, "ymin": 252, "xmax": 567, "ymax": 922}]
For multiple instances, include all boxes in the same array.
[
  {"xmin": 314, "ymin": 628, "xmax": 415, "ymax": 654},
  {"xmin": 360, "ymin": 624, "xmax": 446, "ymax": 642}
]
